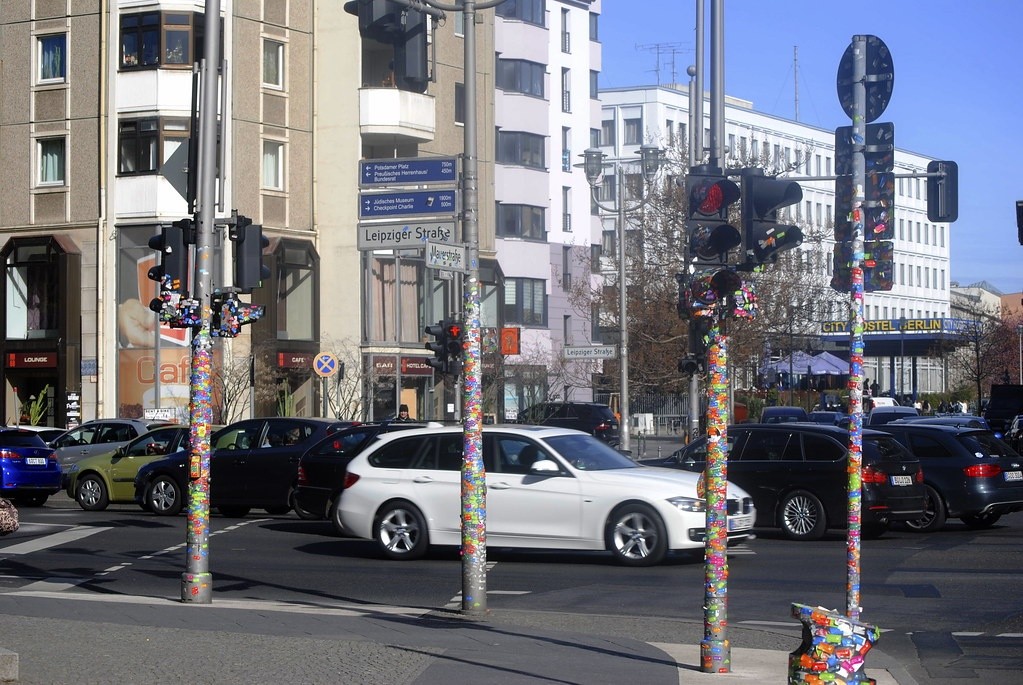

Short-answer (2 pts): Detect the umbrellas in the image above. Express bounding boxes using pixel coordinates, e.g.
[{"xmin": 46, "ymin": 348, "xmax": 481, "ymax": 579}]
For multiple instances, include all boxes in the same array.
[{"xmin": 758, "ymin": 351, "xmax": 849, "ymax": 374}]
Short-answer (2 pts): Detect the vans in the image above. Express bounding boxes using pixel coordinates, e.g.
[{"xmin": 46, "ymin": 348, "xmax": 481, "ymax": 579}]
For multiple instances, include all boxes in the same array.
[{"xmin": 842, "ymin": 396, "xmax": 900, "ymax": 414}]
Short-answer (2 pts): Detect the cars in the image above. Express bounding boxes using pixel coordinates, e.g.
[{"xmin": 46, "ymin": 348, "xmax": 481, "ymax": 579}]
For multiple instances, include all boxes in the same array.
[
  {"xmin": 760, "ymin": 406, "xmax": 844, "ymax": 427},
  {"xmin": 335, "ymin": 424, "xmax": 757, "ymax": 568},
  {"xmin": 64, "ymin": 425, "xmax": 228, "ymax": 512},
  {"xmin": 48, "ymin": 417, "xmax": 175, "ymax": 490},
  {"xmin": 7, "ymin": 425, "xmax": 68, "ymax": 445},
  {"xmin": 0, "ymin": 426, "xmax": 63, "ymax": 509},
  {"xmin": 863, "ymin": 406, "xmax": 1023, "ymax": 456},
  {"xmin": 864, "ymin": 424, "xmax": 1023, "ymax": 533},
  {"xmin": 133, "ymin": 416, "xmax": 367, "ymax": 516},
  {"xmin": 517, "ymin": 401, "xmax": 621, "ymax": 449},
  {"xmin": 638, "ymin": 421, "xmax": 930, "ymax": 541}
]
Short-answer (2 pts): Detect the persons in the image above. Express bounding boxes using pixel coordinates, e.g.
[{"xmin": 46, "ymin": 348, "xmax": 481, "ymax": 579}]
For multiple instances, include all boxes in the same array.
[
  {"xmin": 939, "ymin": 399, "xmax": 968, "ymax": 413},
  {"xmin": 118, "ymin": 226, "xmax": 157, "ymax": 348},
  {"xmin": 905, "ymin": 398, "xmax": 933, "ymax": 415},
  {"xmin": 262, "ymin": 432, "xmax": 271, "ymax": 447},
  {"xmin": 767, "ymin": 382, "xmax": 778, "ymax": 405},
  {"xmin": 864, "ymin": 378, "xmax": 880, "ymax": 396},
  {"xmin": 393, "ymin": 405, "xmax": 412, "ymax": 420},
  {"xmin": 149, "ymin": 438, "xmax": 185, "ymax": 452}
]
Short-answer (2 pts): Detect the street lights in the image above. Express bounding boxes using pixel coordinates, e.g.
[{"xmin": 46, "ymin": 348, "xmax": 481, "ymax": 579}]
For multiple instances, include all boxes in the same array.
[{"xmin": 572, "ymin": 145, "xmax": 671, "ymax": 455}]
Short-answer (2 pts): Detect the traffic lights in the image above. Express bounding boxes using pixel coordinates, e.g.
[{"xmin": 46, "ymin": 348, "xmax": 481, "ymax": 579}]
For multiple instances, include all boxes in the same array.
[
  {"xmin": 424, "ymin": 320, "xmax": 466, "ymax": 376},
  {"xmin": 148, "ymin": 226, "xmax": 187, "ymax": 328},
  {"xmin": 345, "ymin": 0, "xmax": 427, "ymax": 92},
  {"xmin": 236, "ymin": 223, "xmax": 271, "ymax": 294},
  {"xmin": 739, "ymin": 166, "xmax": 804, "ymax": 267},
  {"xmin": 682, "ymin": 164, "xmax": 741, "ymax": 309}
]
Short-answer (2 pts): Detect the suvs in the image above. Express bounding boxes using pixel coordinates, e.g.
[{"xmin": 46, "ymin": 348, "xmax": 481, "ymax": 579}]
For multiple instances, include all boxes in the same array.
[{"xmin": 287, "ymin": 419, "xmax": 463, "ymax": 537}]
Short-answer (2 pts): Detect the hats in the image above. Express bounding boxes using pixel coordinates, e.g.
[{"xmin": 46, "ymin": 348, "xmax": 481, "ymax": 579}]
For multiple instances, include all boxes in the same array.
[{"xmin": 399, "ymin": 405, "xmax": 408, "ymax": 412}]
